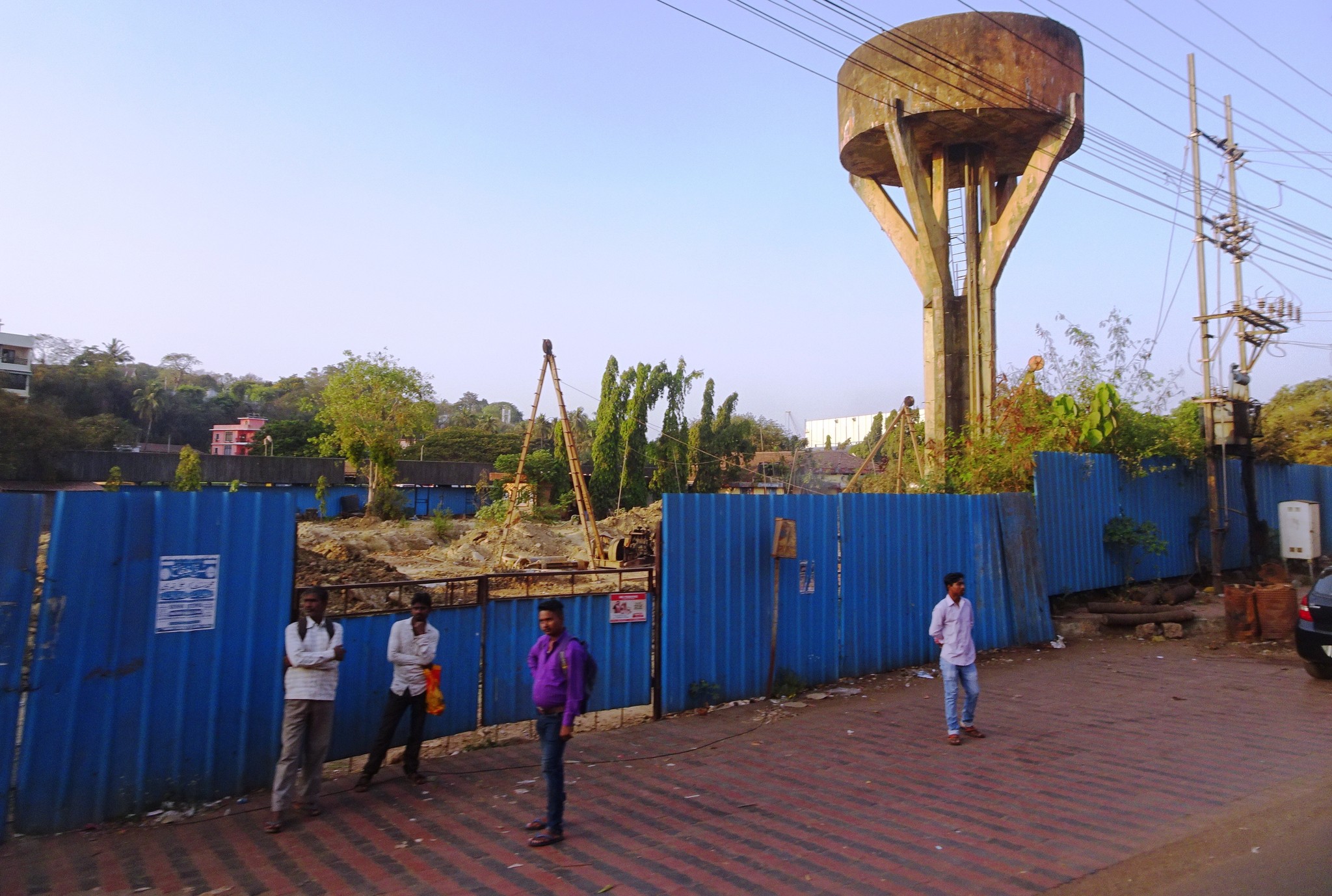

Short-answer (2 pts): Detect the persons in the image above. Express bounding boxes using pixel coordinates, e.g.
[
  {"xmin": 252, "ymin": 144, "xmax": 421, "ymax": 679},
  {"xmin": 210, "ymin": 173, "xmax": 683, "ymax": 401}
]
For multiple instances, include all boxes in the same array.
[
  {"xmin": 929, "ymin": 572, "xmax": 986, "ymax": 745},
  {"xmin": 526, "ymin": 600, "xmax": 586, "ymax": 847},
  {"xmin": 355, "ymin": 592, "xmax": 440, "ymax": 791},
  {"xmin": 265, "ymin": 585, "xmax": 345, "ymax": 832}
]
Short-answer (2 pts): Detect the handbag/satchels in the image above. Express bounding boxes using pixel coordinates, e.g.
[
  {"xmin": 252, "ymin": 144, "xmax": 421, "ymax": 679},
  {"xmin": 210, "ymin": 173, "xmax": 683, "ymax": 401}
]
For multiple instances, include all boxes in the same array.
[{"xmin": 423, "ymin": 665, "xmax": 445, "ymax": 716}]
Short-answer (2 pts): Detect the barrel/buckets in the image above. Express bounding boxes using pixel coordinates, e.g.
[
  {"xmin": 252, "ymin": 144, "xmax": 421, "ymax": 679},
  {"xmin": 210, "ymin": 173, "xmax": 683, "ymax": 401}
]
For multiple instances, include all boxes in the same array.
[
  {"xmin": 1255, "ymin": 581, "xmax": 1299, "ymax": 640},
  {"xmin": 1225, "ymin": 584, "xmax": 1258, "ymax": 644}
]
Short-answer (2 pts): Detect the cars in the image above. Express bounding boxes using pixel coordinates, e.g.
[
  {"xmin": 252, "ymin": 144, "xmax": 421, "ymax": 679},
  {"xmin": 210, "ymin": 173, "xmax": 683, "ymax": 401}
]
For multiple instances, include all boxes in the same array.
[{"xmin": 1295, "ymin": 564, "xmax": 1332, "ymax": 679}]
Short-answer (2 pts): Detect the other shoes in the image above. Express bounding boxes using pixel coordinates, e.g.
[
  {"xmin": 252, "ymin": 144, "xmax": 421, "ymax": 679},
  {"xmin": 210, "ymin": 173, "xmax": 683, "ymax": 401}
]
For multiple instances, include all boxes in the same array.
[
  {"xmin": 948, "ymin": 734, "xmax": 961, "ymax": 746},
  {"xmin": 406, "ymin": 771, "xmax": 427, "ymax": 784},
  {"xmin": 962, "ymin": 727, "xmax": 984, "ymax": 738},
  {"xmin": 357, "ymin": 772, "xmax": 372, "ymax": 791}
]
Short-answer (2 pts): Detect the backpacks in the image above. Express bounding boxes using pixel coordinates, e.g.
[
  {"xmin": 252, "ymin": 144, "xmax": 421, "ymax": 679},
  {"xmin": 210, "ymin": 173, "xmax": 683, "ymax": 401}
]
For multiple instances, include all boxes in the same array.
[{"xmin": 561, "ymin": 638, "xmax": 596, "ymax": 714}]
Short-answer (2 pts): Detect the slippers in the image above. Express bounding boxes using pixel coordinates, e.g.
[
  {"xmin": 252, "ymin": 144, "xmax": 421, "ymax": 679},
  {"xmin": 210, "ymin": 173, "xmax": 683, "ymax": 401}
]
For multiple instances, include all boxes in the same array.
[
  {"xmin": 525, "ymin": 818, "xmax": 548, "ymax": 830},
  {"xmin": 528, "ymin": 833, "xmax": 564, "ymax": 846},
  {"xmin": 263, "ymin": 818, "xmax": 285, "ymax": 833},
  {"xmin": 307, "ymin": 802, "xmax": 323, "ymax": 815}
]
